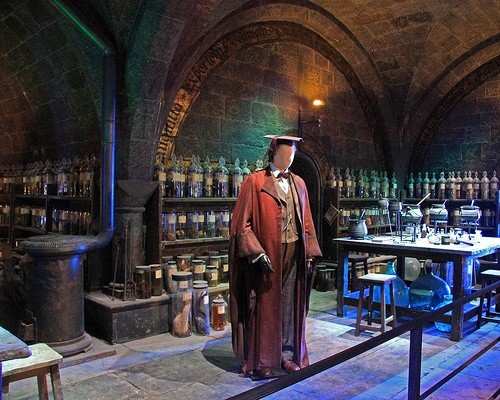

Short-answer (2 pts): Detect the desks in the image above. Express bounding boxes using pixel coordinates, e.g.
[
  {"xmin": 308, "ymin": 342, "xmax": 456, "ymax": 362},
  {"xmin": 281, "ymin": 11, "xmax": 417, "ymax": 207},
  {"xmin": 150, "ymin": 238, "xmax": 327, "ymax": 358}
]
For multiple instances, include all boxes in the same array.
[{"xmin": 333, "ymin": 231, "xmax": 500, "ymax": 341}]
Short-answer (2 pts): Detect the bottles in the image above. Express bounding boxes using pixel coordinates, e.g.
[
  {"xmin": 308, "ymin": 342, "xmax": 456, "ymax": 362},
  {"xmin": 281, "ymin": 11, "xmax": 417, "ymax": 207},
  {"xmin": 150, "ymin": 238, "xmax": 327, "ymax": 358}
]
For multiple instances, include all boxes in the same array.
[
  {"xmin": 157, "ymin": 154, "xmax": 268, "ymax": 199},
  {"xmin": 409, "ymin": 259, "xmax": 451, "ymax": 311},
  {"xmin": 433, "ymin": 294, "xmax": 453, "ymax": 333},
  {"xmin": 339, "ymin": 206, "xmax": 396, "ymax": 240},
  {"xmin": 402, "ymin": 170, "xmax": 499, "ymax": 245},
  {"xmin": 135, "ymin": 250, "xmax": 231, "ymax": 338},
  {"xmin": 0, "ymin": 202, "xmax": 90, "ymax": 236},
  {"xmin": 162, "ymin": 209, "xmax": 233, "ymax": 241},
  {"xmin": 328, "ymin": 166, "xmax": 398, "ymax": 199},
  {"xmin": 418, "ymin": 262, "xmax": 425, "ymax": 277},
  {"xmin": 373, "ymin": 262, "xmax": 409, "ymax": 307},
  {"xmin": 0, "ymin": 154, "xmax": 97, "ymax": 199}
]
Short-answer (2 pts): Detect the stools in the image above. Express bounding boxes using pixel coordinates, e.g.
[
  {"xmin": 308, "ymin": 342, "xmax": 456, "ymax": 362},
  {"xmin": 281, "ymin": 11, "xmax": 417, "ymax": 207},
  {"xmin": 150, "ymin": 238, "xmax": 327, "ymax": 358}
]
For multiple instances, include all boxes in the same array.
[
  {"xmin": 1, "ymin": 343, "xmax": 64, "ymax": 400},
  {"xmin": 477, "ymin": 268, "xmax": 500, "ymax": 328},
  {"xmin": 347, "ymin": 255, "xmax": 369, "ymax": 292},
  {"xmin": 354, "ymin": 272, "xmax": 396, "ymax": 335}
]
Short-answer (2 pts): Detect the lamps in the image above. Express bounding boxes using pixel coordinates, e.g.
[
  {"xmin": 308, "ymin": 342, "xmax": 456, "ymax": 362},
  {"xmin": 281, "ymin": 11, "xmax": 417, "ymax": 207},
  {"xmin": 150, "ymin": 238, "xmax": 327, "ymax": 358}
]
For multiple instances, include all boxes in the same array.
[{"xmin": 298, "ymin": 96, "xmax": 325, "ymax": 137}]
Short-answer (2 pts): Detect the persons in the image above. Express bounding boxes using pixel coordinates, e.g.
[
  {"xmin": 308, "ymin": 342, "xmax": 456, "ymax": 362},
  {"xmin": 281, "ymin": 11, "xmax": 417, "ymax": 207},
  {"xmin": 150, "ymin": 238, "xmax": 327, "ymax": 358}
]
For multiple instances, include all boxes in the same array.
[{"xmin": 229, "ymin": 135, "xmax": 322, "ymax": 379}]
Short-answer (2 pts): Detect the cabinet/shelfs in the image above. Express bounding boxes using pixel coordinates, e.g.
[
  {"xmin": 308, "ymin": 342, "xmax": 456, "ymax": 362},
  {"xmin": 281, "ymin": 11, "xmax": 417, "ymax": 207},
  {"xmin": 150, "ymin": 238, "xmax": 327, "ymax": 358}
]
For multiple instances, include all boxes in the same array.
[
  {"xmin": 325, "ymin": 187, "xmax": 398, "ymax": 265},
  {"xmin": 147, "ymin": 175, "xmax": 238, "ymax": 264},
  {"xmin": 401, "ymin": 190, "xmax": 500, "ymax": 267},
  {"xmin": 0, "ymin": 153, "xmax": 100, "ymax": 250}
]
